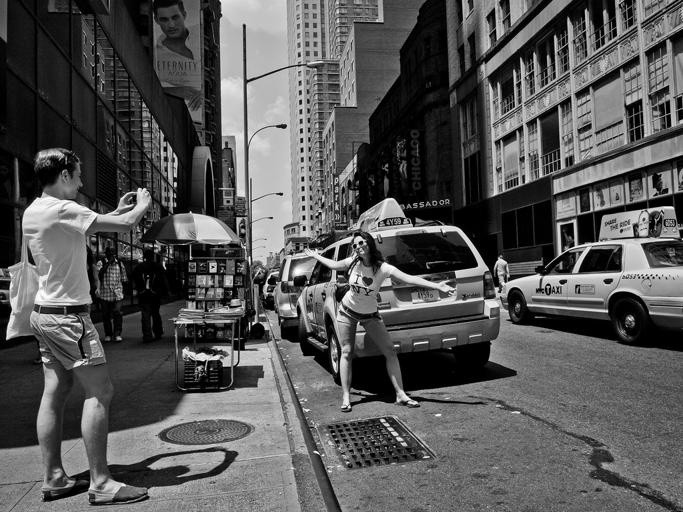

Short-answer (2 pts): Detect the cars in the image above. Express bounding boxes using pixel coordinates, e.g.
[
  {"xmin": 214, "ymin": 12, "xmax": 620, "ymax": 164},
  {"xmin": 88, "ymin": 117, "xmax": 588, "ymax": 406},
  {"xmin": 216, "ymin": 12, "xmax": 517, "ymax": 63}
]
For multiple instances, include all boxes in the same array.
[
  {"xmin": 496, "ymin": 236, "xmax": 682, "ymax": 347},
  {"xmin": 260, "ymin": 268, "xmax": 281, "ymax": 308}
]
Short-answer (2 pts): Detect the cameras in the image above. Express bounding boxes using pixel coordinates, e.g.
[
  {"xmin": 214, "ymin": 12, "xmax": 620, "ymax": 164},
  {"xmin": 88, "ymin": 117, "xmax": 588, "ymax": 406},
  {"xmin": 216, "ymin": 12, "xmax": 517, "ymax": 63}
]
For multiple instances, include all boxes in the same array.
[{"xmin": 130, "ymin": 194, "xmax": 137, "ymax": 203}]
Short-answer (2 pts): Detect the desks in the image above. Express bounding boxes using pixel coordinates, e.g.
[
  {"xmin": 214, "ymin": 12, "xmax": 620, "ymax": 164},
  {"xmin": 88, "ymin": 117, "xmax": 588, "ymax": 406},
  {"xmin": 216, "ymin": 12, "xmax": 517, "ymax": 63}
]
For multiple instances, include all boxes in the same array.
[{"xmin": 171, "ymin": 303, "xmax": 245, "ymax": 391}]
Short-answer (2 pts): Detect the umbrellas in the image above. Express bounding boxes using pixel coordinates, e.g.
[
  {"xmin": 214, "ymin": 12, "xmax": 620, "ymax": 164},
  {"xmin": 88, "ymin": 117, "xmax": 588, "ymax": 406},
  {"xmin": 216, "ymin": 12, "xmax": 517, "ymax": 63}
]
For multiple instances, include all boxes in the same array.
[{"xmin": 139, "ymin": 210, "xmax": 242, "ymax": 257}]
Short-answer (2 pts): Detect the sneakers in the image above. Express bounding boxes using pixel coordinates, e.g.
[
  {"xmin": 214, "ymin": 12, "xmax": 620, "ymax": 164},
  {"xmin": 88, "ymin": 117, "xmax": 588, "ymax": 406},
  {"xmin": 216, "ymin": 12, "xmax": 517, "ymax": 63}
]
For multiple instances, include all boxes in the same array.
[
  {"xmin": 40, "ymin": 479, "xmax": 89, "ymax": 502},
  {"xmin": 104, "ymin": 336, "xmax": 123, "ymax": 341},
  {"xmin": 87, "ymin": 484, "xmax": 148, "ymax": 504}
]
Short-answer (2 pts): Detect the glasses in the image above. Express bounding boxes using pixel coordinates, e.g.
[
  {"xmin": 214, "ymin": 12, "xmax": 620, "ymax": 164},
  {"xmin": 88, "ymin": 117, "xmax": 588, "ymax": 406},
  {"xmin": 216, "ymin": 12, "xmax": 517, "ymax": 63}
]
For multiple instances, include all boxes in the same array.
[{"xmin": 351, "ymin": 240, "xmax": 365, "ymax": 249}]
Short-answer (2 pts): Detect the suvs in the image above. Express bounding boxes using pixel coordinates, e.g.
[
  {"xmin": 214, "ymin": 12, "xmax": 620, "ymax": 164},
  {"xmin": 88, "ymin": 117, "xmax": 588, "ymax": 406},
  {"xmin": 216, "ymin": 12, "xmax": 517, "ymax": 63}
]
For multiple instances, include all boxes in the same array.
[
  {"xmin": 269, "ymin": 246, "xmax": 324, "ymax": 330},
  {"xmin": 296, "ymin": 198, "xmax": 504, "ymax": 385}
]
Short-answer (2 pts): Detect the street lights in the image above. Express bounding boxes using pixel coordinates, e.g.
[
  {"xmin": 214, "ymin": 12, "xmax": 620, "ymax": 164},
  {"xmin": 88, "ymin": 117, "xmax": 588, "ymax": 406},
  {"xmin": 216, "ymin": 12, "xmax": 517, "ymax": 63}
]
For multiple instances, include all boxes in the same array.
[
  {"xmin": 249, "ymin": 178, "xmax": 285, "ymax": 222},
  {"xmin": 243, "ymin": 58, "xmax": 326, "ymax": 308},
  {"xmin": 249, "ymin": 119, "xmax": 290, "ymax": 150},
  {"xmin": 248, "ymin": 214, "xmax": 274, "ymax": 226},
  {"xmin": 252, "ymin": 245, "xmax": 266, "ymax": 252},
  {"xmin": 251, "ymin": 237, "xmax": 268, "ymax": 243}
]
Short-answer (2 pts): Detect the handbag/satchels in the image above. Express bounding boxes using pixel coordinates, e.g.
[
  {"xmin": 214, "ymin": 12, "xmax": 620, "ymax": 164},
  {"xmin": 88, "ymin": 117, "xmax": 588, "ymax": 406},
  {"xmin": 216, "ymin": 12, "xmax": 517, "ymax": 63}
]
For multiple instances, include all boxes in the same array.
[
  {"xmin": 5, "ymin": 237, "xmax": 39, "ymax": 341},
  {"xmin": 334, "ymin": 284, "xmax": 349, "ymax": 303}
]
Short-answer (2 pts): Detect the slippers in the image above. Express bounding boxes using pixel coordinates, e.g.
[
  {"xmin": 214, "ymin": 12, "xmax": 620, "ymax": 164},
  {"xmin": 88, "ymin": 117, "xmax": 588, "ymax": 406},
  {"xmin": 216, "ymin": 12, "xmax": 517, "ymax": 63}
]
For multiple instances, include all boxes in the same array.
[
  {"xmin": 393, "ymin": 399, "xmax": 419, "ymax": 408},
  {"xmin": 341, "ymin": 403, "xmax": 351, "ymax": 411}
]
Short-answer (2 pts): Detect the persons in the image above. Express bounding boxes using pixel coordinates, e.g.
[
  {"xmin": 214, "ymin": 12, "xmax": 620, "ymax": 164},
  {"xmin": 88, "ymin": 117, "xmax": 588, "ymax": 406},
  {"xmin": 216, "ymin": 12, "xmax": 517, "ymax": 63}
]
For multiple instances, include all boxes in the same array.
[
  {"xmin": 133, "ymin": 247, "xmax": 166, "ymax": 344},
  {"xmin": 86, "ymin": 244, "xmax": 100, "ymax": 325},
  {"xmin": 153, "ymin": 0, "xmax": 204, "ymax": 146},
  {"xmin": 303, "ymin": 231, "xmax": 457, "ymax": 412},
  {"xmin": 653, "ymin": 172, "xmax": 668, "ymax": 197},
  {"xmin": 494, "ymin": 254, "xmax": 510, "ymax": 293},
  {"xmin": 98, "ymin": 247, "xmax": 128, "ymax": 342},
  {"xmin": 22, "ymin": 147, "xmax": 152, "ymax": 504}
]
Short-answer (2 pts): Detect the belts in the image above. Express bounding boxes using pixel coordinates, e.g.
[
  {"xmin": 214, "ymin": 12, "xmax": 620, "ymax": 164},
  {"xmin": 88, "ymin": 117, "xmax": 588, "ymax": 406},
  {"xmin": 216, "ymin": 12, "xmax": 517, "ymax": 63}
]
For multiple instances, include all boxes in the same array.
[
  {"xmin": 34, "ymin": 304, "xmax": 88, "ymax": 314},
  {"xmin": 343, "ymin": 306, "xmax": 380, "ymax": 320}
]
